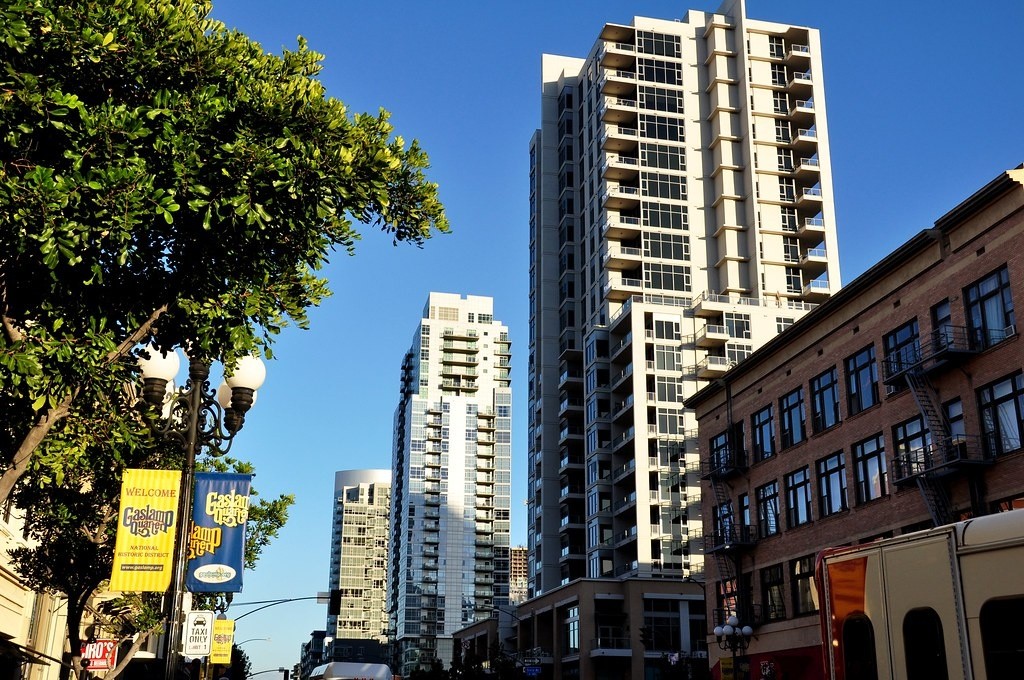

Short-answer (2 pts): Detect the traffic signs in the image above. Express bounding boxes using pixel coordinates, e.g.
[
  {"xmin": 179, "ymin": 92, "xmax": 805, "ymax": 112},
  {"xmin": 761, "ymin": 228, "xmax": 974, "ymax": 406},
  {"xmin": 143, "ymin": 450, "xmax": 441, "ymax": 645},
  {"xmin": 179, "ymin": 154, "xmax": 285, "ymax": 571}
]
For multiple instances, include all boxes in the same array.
[{"xmin": 523, "ymin": 656, "xmax": 542, "ymax": 666}]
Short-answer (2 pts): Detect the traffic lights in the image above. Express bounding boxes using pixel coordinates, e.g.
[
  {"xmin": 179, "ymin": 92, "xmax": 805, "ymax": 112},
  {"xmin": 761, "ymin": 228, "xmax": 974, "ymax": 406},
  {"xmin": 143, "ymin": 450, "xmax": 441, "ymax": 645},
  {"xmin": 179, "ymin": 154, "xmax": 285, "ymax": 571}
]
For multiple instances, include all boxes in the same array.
[{"xmin": 639, "ymin": 625, "xmax": 654, "ymax": 651}]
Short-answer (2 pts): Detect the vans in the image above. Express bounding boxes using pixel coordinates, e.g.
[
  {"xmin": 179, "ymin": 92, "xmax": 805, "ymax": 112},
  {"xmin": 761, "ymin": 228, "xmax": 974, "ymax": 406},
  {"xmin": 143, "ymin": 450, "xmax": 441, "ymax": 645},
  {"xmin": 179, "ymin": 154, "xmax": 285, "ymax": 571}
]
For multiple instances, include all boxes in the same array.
[{"xmin": 308, "ymin": 662, "xmax": 392, "ymax": 680}]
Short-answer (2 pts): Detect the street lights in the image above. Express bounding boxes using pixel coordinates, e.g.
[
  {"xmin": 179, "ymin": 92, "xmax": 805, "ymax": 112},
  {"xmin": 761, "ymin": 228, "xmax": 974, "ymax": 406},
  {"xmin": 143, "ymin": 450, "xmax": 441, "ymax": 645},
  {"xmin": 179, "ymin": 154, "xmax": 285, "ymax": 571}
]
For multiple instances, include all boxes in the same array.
[
  {"xmin": 714, "ymin": 616, "xmax": 753, "ymax": 680},
  {"xmin": 134, "ymin": 336, "xmax": 266, "ymax": 680}
]
